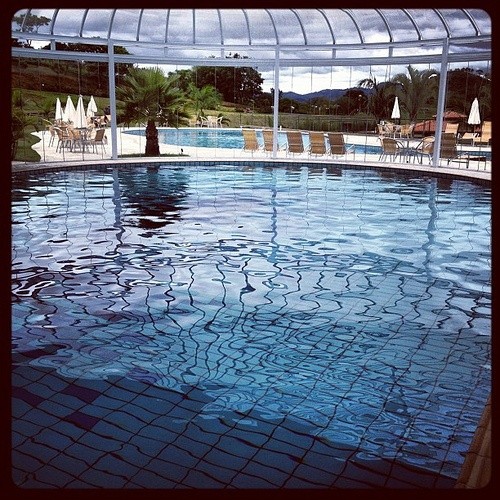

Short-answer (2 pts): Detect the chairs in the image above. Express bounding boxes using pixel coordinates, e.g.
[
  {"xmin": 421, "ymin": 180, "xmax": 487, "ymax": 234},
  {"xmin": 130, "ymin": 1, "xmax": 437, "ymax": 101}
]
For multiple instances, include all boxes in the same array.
[
  {"xmin": 198, "ymin": 114, "xmax": 492, "ymax": 170},
  {"xmin": 44, "ymin": 113, "xmax": 112, "ymax": 154}
]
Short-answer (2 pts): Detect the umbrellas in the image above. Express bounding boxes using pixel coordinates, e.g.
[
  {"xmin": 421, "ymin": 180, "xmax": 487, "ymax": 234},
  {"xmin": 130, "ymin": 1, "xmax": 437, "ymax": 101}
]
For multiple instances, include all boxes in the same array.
[
  {"xmin": 469, "ymin": 97, "xmax": 480, "ymax": 146},
  {"xmin": 390, "ymin": 96, "xmax": 402, "ymax": 125},
  {"xmin": 55, "ymin": 93, "xmax": 98, "ymax": 134}
]
